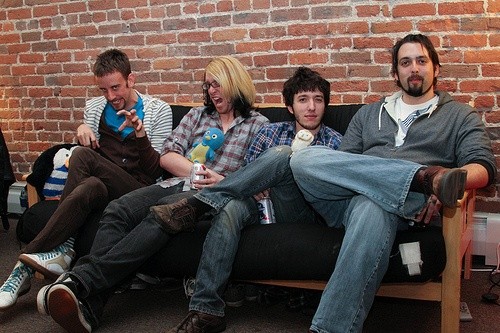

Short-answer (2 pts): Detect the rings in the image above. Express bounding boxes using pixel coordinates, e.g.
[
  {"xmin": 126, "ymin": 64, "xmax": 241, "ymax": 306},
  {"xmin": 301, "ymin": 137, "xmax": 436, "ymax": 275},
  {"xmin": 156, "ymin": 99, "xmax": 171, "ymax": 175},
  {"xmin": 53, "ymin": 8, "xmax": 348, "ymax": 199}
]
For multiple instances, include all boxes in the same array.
[{"xmin": 432, "ymin": 199, "xmax": 437, "ymax": 205}]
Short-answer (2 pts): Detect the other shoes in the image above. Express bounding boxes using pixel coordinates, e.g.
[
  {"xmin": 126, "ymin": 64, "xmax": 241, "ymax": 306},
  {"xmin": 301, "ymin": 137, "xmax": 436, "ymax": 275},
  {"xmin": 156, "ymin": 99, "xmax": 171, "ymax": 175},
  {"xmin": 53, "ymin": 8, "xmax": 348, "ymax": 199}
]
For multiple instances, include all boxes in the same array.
[
  {"xmin": 419, "ymin": 166, "xmax": 468, "ymax": 208},
  {"xmin": 188, "ymin": 280, "xmax": 260, "ymax": 308}
]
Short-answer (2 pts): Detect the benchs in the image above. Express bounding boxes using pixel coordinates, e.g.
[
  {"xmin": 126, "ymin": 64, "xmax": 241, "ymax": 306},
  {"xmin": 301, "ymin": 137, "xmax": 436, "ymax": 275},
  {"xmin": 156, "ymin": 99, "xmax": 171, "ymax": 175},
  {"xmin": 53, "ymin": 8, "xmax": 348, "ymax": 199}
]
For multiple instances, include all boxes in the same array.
[{"xmin": 21, "ymin": 101, "xmax": 476, "ymax": 333}]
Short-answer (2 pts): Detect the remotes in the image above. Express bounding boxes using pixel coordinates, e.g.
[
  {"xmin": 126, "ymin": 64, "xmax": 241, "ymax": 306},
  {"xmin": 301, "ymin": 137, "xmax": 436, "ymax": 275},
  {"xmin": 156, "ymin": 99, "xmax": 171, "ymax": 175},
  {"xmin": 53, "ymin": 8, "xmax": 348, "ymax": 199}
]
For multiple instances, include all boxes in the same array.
[{"xmin": 459, "ymin": 302, "xmax": 472, "ymax": 322}]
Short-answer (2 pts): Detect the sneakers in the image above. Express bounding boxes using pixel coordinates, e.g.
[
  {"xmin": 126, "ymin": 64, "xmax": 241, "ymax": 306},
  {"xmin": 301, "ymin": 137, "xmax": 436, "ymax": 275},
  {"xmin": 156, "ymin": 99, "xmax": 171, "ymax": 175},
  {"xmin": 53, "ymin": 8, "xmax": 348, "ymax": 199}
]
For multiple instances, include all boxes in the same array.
[
  {"xmin": 149, "ymin": 198, "xmax": 199, "ymax": 233},
  {"xmin": 164, "ymin": 310, "xmax": 227, "ymax": 333},
  {"xmin": 18, "ymin": 245, "xmax": 75, "ymax": 281},
  {"xmin": 36, "ymin": 272, "xmax": 78, "ymax": 319},
  {"xmin": 45, "ymin": 283, "xmax": 95, "ymax": 333},
  {"xmin": 0, "ymin": 260, "xmax": 33, "ymax": 309}
]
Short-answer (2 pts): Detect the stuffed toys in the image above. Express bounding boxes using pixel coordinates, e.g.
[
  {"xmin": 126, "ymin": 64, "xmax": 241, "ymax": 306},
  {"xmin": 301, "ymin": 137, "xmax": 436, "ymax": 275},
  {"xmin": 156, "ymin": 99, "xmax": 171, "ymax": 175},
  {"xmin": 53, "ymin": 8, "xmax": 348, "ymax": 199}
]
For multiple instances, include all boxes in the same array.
[
  {"xmin": 43, "ymin": 147, "xmax": 80, "ymax": 201},
  {"xmin": 187, "ymin": 127, "xmax": 225, "ymax": 163}
]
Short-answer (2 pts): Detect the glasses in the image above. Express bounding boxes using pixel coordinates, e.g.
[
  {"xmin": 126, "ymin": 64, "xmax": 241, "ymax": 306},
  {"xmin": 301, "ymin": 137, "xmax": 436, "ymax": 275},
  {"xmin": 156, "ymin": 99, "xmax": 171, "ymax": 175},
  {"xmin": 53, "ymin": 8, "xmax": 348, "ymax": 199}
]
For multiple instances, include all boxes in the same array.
[{"xmin": 201, "ymin": 81, "xmax": 221, "ymax": 90}]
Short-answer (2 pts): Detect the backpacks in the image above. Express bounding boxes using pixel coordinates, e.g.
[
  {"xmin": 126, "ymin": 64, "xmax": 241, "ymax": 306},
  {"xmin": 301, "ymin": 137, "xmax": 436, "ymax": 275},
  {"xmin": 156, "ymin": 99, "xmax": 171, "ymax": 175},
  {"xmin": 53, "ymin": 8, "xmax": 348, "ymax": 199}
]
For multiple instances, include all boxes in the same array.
[{"xmin": 16, "ymin": 199, "xmax": 60, "ymax": 249}]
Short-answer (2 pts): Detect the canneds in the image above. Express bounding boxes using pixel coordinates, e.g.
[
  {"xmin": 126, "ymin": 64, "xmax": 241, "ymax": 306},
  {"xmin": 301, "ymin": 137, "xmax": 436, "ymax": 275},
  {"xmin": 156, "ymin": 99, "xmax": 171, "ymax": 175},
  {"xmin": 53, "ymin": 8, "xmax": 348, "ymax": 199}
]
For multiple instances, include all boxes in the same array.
[
  {"xmin": 257, "ymin": 196, "xmax": 277, "ymax": 225},
  {"xmin": 190, "ymin": 163, "xmax": 207, "ymax": 189}
]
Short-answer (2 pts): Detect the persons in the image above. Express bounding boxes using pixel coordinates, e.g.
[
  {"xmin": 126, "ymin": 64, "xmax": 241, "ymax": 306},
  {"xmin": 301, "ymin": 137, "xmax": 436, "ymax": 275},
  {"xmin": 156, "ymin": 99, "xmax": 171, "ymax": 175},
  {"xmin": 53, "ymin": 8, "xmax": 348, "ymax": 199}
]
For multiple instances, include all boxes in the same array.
[
  {"xmin": 37, "ymin": 56, "xmax": 271, "ymax": 333},
  {"xmin": 0, "ymin": 49, "xmax": 173, "ymax": 309},
  {"xmin": 289, "ymin": 34, "xmax": 498, "ymax": 333},
  {"xmin": 151, "ymin": 65, "xmax": 345, "ymax": 333}
]
